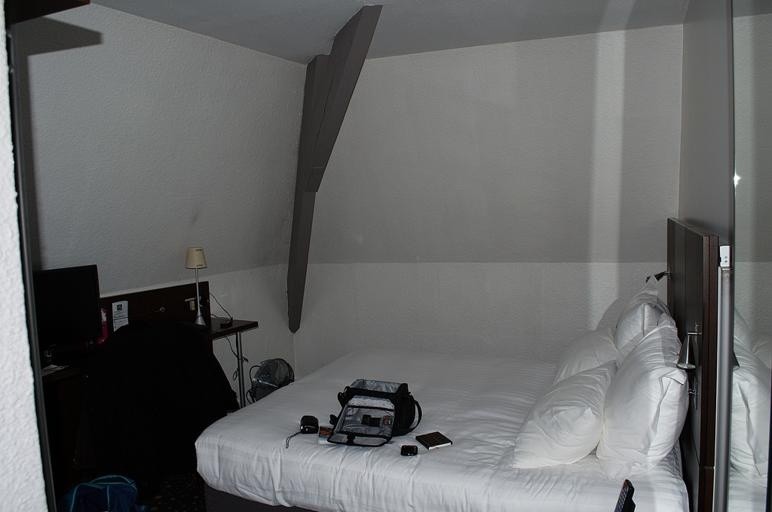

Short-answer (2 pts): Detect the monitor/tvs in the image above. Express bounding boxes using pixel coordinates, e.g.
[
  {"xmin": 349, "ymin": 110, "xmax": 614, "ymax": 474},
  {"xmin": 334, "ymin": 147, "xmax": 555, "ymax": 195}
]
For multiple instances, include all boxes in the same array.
[{"xmin": 33, "ymin": 265, "xmax": 102, "ymax": 377}]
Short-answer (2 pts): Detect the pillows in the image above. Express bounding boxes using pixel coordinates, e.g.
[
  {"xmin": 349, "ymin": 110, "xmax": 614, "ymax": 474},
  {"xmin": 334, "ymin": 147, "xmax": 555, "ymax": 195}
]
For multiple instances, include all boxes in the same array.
[
  {"xmin": 612, "ymin": 269, "xmax": 666, "ymax": 370},
  {"xmin": 510, "ymin": 360, "xmax": 619, "ymax": 469},
  {"xmin": 553, "ymin": 329, "xmax": 620, "ymax": 385},
  {"xmin": 729, "ymin": 303, "xmax": 772, "ymax": 474},
  {"xmin": 595, "ymin": 311, "xmax": 689, "ymax": 475}
]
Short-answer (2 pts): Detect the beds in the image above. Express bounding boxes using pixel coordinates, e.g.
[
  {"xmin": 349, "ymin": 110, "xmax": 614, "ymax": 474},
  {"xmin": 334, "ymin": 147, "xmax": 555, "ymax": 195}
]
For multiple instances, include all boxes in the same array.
[{"xmin": 184, "ymin": 214, "xmax": 719, "ymax": 512}]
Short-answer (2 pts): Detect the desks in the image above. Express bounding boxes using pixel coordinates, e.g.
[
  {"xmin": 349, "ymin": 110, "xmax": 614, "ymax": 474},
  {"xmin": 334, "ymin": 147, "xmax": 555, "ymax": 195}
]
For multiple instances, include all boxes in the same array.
[{"xmin": 55, "ymin": 315, "xmax": 259, "ymax": 511}]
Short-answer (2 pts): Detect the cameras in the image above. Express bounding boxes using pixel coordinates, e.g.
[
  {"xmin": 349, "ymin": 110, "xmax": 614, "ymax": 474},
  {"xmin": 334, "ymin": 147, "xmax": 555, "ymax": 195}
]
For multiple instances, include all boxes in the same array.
[{"xmin": 301, "ymin": 416, "xmax": 318, "ymax": 434}]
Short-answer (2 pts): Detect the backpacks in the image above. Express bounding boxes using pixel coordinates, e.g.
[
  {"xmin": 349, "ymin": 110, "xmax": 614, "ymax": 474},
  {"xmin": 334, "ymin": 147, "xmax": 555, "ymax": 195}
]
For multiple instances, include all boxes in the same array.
[{"xmin": 251, "ymin": 357, "xmax": 295, "ymax": 404}]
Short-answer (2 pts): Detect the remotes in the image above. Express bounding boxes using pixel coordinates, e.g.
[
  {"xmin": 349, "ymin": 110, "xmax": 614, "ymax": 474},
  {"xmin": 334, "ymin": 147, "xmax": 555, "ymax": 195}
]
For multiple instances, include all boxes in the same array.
[
  {"xmin": 220, "ymin": 317, "xmax": 233, "ymax": 327},
  {"xmin": 614, "ymin": 480, "xmax": 634, "ymax": 512}
]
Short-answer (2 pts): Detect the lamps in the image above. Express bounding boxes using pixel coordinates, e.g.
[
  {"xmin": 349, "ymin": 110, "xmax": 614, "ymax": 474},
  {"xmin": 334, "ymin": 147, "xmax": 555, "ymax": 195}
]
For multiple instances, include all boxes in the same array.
[
  {"xmin": 185, "ymin": 246, "xmax": 209, "ymax": 327},
  {"xmin": 674, "ymin": 321, "xmax": 701, "ymax": 409},
  {"xmin": 644, "ymin": 264, "xmax": 672, "ymax": 285}
]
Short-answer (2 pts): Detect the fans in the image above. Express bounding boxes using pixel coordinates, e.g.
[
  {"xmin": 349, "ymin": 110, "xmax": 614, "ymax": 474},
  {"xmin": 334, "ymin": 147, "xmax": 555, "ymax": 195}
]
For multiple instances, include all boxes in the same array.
[{"xmin": 247, "ymin": 355, "xmax": 295, "ymax": 401}]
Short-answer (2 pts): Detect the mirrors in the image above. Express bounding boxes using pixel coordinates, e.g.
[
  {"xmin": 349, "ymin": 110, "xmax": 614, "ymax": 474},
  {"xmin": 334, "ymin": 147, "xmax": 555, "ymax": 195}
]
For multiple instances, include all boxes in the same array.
[{"xmin": 726, "ymin": 2, "xmax": 772, "ymax": 512}]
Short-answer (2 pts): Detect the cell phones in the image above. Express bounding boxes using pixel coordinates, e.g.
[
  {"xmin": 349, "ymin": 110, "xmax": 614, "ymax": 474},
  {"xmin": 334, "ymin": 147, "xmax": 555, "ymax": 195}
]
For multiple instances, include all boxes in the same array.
[{"xmin": 401, "ymin": 445, "xmax": 417, "ymax": 456}]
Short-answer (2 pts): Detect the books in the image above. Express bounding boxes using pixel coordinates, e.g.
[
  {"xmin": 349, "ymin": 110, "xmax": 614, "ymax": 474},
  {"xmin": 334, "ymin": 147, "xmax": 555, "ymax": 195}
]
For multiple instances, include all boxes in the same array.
[
  {"xmin": 416, "ymin": 432, "xmax": 453, "ymax": 451},
  {"xmin": 318, "ymin": 424, "xmax": 336, "ymax": 447}
]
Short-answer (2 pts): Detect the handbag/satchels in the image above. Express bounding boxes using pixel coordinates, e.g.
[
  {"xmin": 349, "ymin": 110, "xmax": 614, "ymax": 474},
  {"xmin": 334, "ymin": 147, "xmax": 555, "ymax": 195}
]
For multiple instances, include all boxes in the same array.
[
  {"xmin": 327, "ymin": 377, "xmax": 424, "ymax": 449},
  {"xmin": 61, "ymin": 474, "xmax": 143, "ymax": 512}
]
